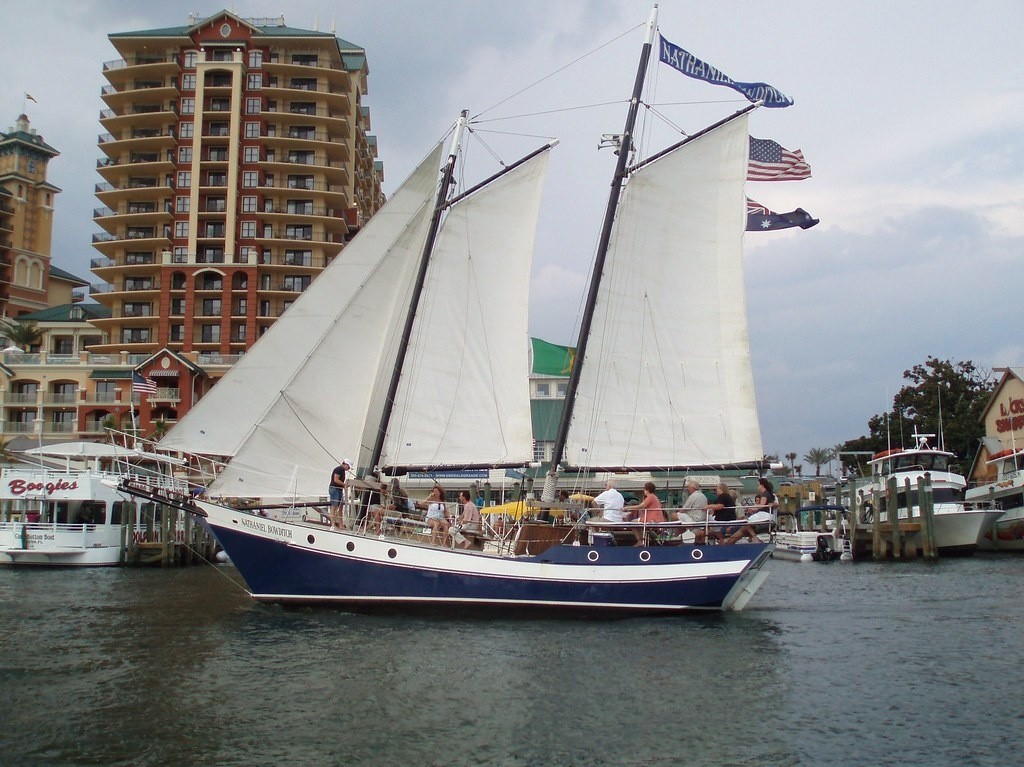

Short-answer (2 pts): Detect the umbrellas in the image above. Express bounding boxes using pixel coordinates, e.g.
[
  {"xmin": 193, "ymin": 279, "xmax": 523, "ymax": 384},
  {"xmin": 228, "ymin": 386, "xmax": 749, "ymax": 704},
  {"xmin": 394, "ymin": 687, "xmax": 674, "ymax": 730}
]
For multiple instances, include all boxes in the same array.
[{"xmin": 568, "ymin": 492, "xmax": 594, "ymax": 510}]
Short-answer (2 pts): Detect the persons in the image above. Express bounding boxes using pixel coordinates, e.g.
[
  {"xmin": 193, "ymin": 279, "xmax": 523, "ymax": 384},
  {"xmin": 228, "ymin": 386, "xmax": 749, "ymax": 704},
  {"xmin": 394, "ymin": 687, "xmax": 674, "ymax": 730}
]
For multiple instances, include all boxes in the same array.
[
  {"xmin": 857, "ymin": 486, "xmax": 874, "ymax": 524},
  {"xmin": 356, "ymin": 478, "xmax": 528, "ymax": 549},
  {"xmin": 329, "ymin": 459, "xmax": 354, "ymax": 531},
  {"xmin": 558, "ymin": 478, "xmax": 779, "ymax": 547}
]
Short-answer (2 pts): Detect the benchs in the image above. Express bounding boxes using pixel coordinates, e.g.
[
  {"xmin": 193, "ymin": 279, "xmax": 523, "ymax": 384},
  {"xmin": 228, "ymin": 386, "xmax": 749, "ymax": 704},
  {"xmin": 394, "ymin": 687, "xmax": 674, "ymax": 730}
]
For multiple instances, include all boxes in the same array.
[
  {"xmin": 456, "ymin": 528, "xmax": 492, "ymax": 545},
  {"xmin": 384, "ymin": 516, "xmax": 429, "ymax": 538}
]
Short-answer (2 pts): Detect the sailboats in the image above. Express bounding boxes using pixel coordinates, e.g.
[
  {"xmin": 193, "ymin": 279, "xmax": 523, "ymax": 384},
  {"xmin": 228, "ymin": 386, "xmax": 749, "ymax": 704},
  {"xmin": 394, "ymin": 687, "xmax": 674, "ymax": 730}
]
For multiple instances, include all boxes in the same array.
[{"xmin": 101, "ymin": 3, "xmax": 822, "ymax": 613}]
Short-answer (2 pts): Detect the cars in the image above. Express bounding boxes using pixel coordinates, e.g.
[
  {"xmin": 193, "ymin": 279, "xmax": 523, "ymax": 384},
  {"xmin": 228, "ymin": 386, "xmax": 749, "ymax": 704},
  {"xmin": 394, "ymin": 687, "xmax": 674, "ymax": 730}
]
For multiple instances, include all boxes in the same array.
[{"xmin": 774, "ymin": 476, "xmax": 847, "ymax": 492}]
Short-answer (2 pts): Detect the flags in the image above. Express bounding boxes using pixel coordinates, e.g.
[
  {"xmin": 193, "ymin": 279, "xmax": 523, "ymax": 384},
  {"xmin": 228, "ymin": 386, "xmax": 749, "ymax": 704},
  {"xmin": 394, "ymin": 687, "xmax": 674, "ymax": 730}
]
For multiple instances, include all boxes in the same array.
[
  {"xmin": 26, "ymin": 93, "xmax": 37, "ymax": 103},
  {"xmin": 747, "ymin": 135, "xmax": 812, "ymax": 182},
  {"xmin": 660, "ymin": 31, "xmax": 794, "ymax": 107},
  {"xmin": 132, "ymin": 371, "xmax": 157, "ymax": 394},
  {"xmin": 745, "ymin": 195, "xmax": 820, "ymax": 231}
]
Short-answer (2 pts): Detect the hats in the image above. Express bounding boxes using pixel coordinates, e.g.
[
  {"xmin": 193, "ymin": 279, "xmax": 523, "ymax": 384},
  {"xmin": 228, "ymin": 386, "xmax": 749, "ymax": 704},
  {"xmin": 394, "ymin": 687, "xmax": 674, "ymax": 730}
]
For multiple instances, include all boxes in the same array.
[{"xmin": 342, "ymin": 459, "xmax": 353, "ymax": 470}]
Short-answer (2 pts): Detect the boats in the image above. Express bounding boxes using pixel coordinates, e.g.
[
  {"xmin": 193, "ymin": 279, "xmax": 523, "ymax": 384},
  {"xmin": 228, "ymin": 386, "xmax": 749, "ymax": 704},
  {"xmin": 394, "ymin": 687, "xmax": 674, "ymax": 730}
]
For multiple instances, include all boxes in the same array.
[
  {"xmin": 827, "ymin": 386, "xmax": 1007, "ymax": 559},
  {"xmin": 0, "ymin": 371, "xmax": 222, "ymax": 567},
  {"xmin": 771, "ymin": 505, "xmax": 854, "ymax": 562},
  {"xmin": 964, "ymin": 408, "xmax": 1024, "ymax": 551}
]
